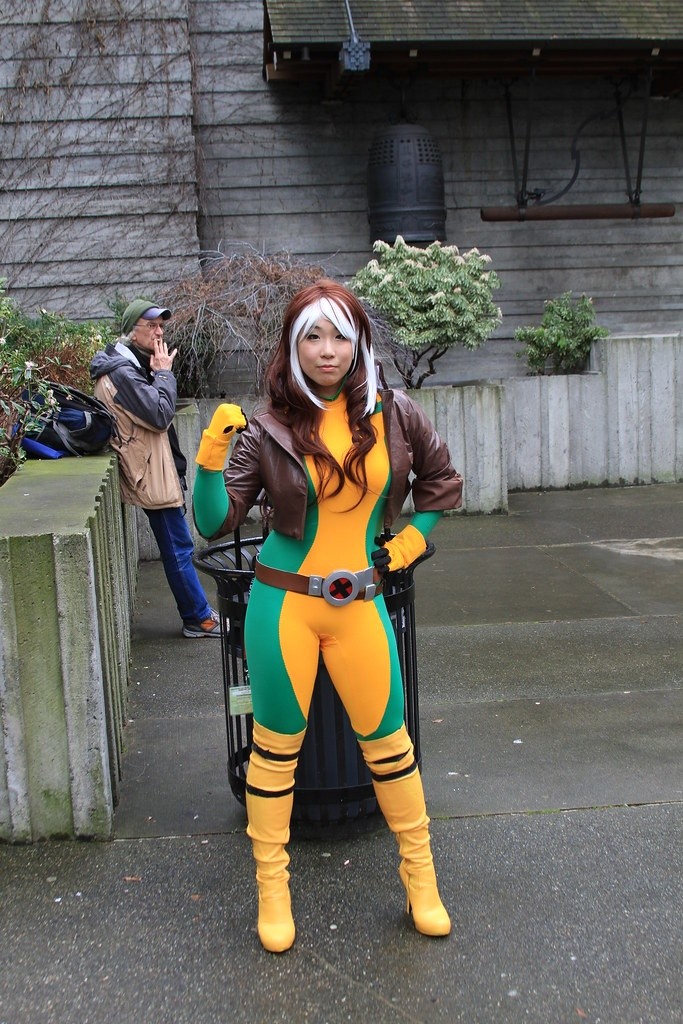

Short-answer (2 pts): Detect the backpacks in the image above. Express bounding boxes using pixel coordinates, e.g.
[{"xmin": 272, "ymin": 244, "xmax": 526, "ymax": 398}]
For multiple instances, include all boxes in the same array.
[{"xmin": 12, "ymin": 378, "xmax": 123, "ymax": 461}]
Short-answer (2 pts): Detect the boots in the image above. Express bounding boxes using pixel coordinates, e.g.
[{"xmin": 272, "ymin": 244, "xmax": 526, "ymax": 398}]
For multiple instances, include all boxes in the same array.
[
  {"xmin": 245, "ymin": 789, "xmax": 296, "ymax": 953},
  {"xmin": 371, "ymin": 764, "xmax": 452, "ymax": 937}
]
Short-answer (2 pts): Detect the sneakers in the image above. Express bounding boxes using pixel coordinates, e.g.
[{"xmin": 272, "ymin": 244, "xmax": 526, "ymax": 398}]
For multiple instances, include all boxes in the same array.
[{"xmin": 182, "ymin": 608, "xmax": 231, "ymax": 638}]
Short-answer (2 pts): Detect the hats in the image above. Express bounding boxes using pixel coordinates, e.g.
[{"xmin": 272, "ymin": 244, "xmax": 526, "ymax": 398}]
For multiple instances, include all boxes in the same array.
[{"xmin": 121, "ymin": 299, "xmax": 172, "ymax": 336}]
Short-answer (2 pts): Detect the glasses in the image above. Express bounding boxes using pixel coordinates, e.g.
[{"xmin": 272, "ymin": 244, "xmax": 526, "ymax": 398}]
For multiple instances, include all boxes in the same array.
[{"xmin": 132, "ymin": 321, "xmax": 166, "ymax": 329}]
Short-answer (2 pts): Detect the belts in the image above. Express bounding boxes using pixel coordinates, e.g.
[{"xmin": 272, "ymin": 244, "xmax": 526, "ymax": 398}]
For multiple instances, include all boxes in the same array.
[{"xmin": 252, "ymin": 553, "xmax": 387, "ymax": 608}]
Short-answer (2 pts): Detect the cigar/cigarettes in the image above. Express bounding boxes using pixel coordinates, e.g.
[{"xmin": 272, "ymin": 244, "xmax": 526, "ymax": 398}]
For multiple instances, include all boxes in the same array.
[{"xmin": 153, "ymin": 337, "xmax": 161, "ymax": 343}]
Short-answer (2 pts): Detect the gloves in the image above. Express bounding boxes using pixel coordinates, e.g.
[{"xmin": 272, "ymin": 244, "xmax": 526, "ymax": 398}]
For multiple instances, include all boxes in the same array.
[
  {"xmin": 194, "ymin": 403, "xmax": 249, "ymax": 472},
  {"xmin": 370, "ymin": 525, "xmax": 427, "ymax": 576}
]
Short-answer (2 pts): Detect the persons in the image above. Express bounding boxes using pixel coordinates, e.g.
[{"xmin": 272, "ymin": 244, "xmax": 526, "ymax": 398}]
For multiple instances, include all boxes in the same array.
[
  {"xmin": 86, "ymin": 298, "xmax": 231, "ymax": 647},
  {"xmin": 193, "ymin": 280, "xmax": 464, "ymax": 954}
]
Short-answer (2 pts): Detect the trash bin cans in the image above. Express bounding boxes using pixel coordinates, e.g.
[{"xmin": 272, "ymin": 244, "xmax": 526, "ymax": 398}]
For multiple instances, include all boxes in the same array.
[{"xmin": 194, "ymin": 498, "xmax": 437, "ymax": 829}]
